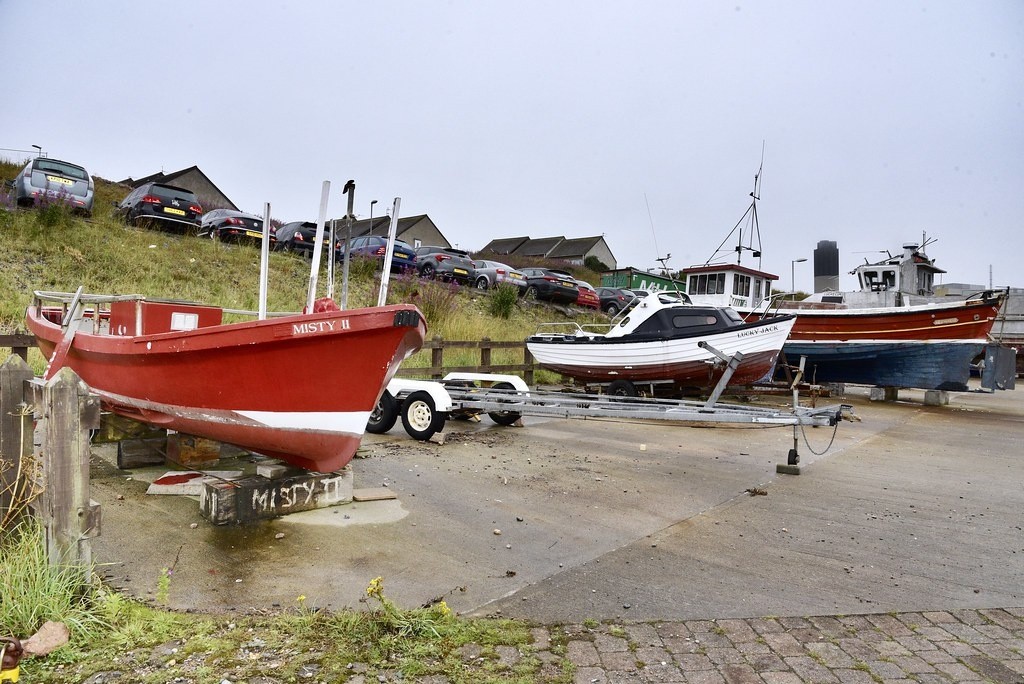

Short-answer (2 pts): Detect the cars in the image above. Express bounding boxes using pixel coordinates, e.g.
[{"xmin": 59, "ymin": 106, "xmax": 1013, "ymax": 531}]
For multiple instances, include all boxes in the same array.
[
  {"xmin": 517, "ymin": 267, "xmax": 579, "ymax": 306},
  {"xmin": 415, "ymin": 246, "xmax": 476, "ymax": 286},
  {"xmin": 7, "ymin": 158, "xmax": 95, "ymax": 219},
  {"xmin": 111, "ymin": 182, "xmax": 204, "ymax": 236},
  {"xmin": 594, "ymin": 287, "xmax": 641, "ymax": 314},
  {"xmin": 569, "ymin": 280, "xmax": 601, "ymax": 310},
  {"xmin": 632, "ymin": 289, "xmax": 654, "ymax": 301},
  {"xmin": 276, "ymin": 221, "xmax": 344, "ymax": 260},
  {"xmin": 335, "ymin": 236, "xmax": 419, "ymax": 275},
  {"xmin": 198, "ymin": 209, "xmax": 278, "ymax": 250},
  {"xmin": 470, "ymin": 260, "xmax": 529, "ymax": 296}
]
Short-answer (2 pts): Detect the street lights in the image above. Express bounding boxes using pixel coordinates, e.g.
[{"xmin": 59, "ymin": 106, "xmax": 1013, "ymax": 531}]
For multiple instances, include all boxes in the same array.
[
  {"xmin": 32, "ymin": 145, "xmax": 42, "ymax": 158},
  {"xmin": 791, "ymin": 258, "xmax": 817, "ymax": 301},
  {"xmin": 370, "ymin": 199, "xmax": 378, "ymax": 234}
]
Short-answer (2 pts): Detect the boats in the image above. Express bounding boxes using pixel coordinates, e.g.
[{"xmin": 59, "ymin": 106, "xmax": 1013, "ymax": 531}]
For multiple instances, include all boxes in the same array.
[
  {"xmin": 533, "ymin": 293, "xmax": 799, "ymax": 390},
  {"xmin": 707, "ymin": 286, "xmax": 1005, "ymax": 393},
  {"xmin": 24, "ymin": 289, "xmax": 430, "ymax": 472}
]
